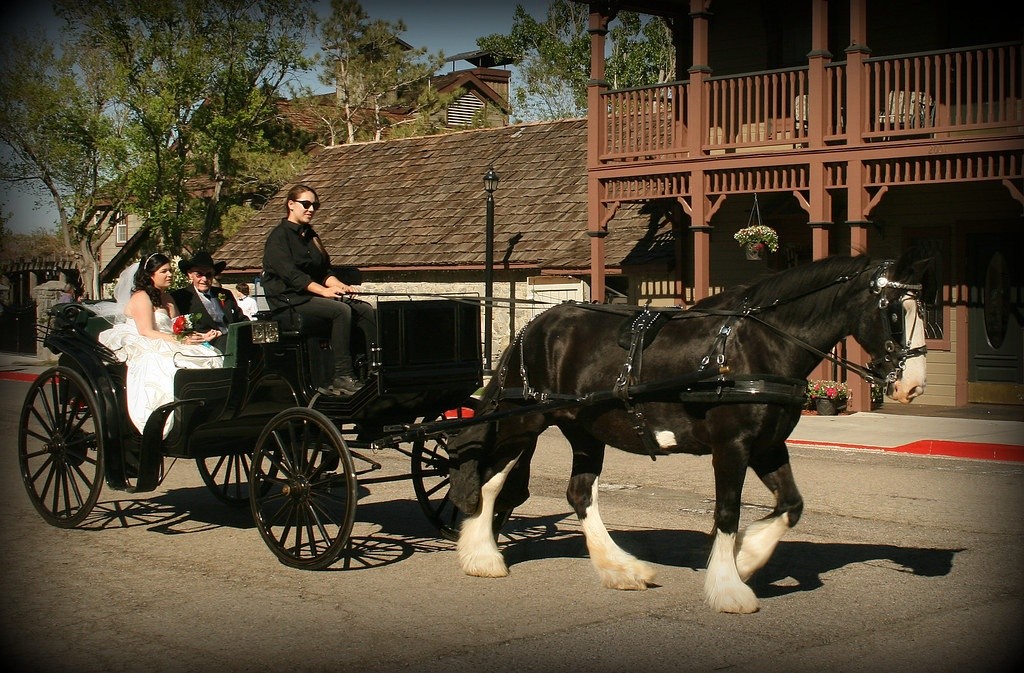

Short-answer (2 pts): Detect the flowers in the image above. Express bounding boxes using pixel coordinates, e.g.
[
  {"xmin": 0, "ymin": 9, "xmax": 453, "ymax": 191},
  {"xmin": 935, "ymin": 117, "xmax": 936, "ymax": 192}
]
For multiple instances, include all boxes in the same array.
[
  {"xmin": 734, "ymin": 226, "xmax": 779, "ymax": 254},
  {"xmin": 806, "ymin": 380, "xmax": 847, "ymax": 401},
  {"xmin": 218, "ymin": 293, "xmax": 226, "ymax": 306},
  {"xmin": 173, "ymin": 312, "xmax": 202, "ymax": 341}
]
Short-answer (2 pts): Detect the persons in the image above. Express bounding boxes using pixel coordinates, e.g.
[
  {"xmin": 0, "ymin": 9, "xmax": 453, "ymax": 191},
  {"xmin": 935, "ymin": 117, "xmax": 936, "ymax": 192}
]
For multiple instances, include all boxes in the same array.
[
  {"xmin": 263, "ymin": 185, "xmax": 374, "ymax": 395},
  {"xmin": 170, "ymin": 251, "xmax": 250, "ymax": 354},
  {"xmin": 83, "ymin": 252, "xmax": 225, "ymax": 440},
  {"xmin": 235, "ymin": 283, "xmax": 259, "ymax": 321}
]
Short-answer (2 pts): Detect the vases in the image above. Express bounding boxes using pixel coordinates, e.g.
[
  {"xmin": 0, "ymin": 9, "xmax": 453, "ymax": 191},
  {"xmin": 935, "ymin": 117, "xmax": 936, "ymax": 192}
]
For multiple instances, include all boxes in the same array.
[
  {"xmin": 815, "ymin": 396, "xmax": 837, "ymax": 415},
  {"xmin": 745, "ymin": 242, "xmax": 763, "ymax": 260}
]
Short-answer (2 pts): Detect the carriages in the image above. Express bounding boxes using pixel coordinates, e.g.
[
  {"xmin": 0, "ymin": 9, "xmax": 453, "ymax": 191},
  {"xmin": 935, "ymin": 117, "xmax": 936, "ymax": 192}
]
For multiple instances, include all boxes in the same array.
[{"xmin": 18, "ymin": 252, "xmax": 937, "ymax": 614}]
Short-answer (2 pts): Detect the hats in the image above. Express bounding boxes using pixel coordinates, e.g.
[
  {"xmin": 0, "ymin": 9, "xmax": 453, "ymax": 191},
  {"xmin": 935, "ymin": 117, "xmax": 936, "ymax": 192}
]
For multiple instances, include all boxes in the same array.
[{"xmin": 178, "ymin": 249, "xmax": 226, "ymax": 276}]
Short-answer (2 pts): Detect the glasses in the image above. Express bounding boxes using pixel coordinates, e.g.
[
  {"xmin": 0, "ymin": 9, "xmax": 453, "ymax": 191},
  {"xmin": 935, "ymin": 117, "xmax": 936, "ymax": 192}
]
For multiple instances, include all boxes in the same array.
[
  {"xmin": 291, "ymin": 199, "xmax": 321, "ymax": 210},
  {"xmin": 190, "ymin": 270, "xmax": 214, "ymax": 277}
]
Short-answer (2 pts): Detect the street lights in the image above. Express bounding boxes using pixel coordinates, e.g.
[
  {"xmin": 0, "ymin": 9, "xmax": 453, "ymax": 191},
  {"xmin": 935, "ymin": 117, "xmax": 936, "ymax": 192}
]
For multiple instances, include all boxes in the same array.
[{"xmin": 482, "ymin": 162, "xmax": 498, "ymax": 371}]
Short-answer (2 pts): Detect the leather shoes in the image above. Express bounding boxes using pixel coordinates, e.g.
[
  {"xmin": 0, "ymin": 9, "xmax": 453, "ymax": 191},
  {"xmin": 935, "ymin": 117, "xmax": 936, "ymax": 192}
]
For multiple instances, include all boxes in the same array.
[{"xmin": 333, "ymin": 376, "xmax": 365, "ymax": 396}]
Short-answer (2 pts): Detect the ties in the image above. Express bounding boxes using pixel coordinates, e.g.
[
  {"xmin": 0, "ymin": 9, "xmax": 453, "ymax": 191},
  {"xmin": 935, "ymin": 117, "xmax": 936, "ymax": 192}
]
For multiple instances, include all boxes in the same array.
[{"xmin": 203, "ymin": 292, "xmax": 211, "ymax": 300}]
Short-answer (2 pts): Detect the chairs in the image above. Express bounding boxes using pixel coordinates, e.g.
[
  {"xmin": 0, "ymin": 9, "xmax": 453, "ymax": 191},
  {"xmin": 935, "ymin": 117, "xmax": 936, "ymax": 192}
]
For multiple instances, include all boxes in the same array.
[
  {"xmin": 795, "ymin": 95, "xmax": 808, "ymax": 138},
  {"xmin": 879, "ymin": 90, "xmax": 936, "ymax": 142}
]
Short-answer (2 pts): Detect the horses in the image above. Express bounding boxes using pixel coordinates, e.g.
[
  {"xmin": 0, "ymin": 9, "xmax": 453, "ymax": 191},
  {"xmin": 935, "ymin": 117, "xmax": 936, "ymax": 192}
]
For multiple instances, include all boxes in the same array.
[{"xmin": 454, "ymin": 244, "xmax": 942, "ymax": 617}]
